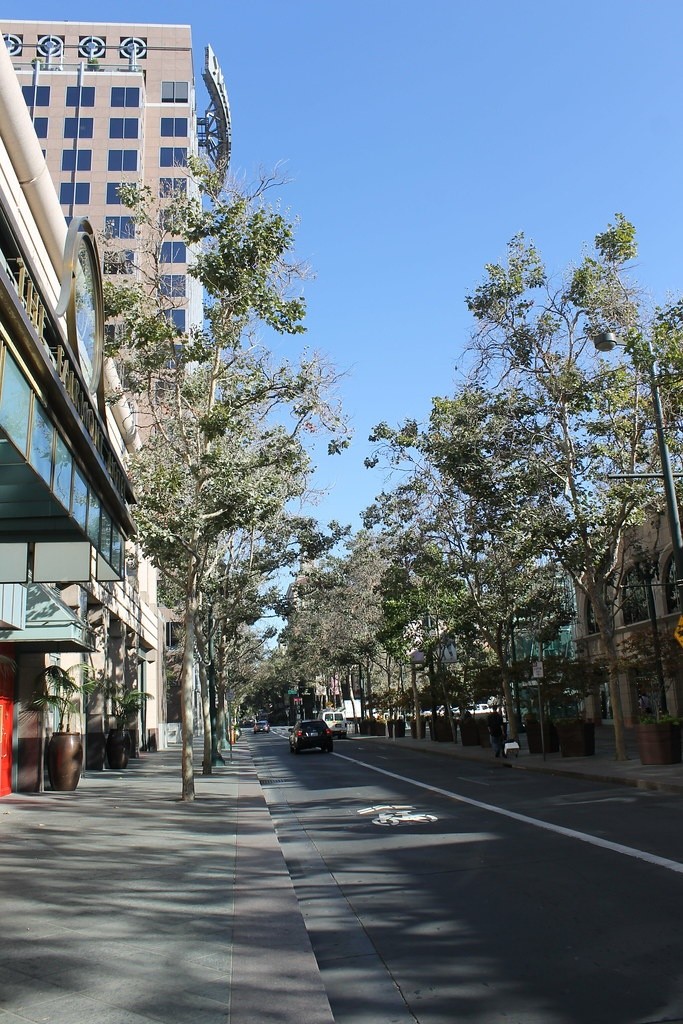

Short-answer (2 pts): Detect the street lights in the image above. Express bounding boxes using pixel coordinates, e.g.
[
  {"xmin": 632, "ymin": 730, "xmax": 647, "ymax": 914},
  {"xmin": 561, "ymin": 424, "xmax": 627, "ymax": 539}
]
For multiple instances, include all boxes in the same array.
[{"xmin": 594, "ymin": 331, "xmax": 683, "ymax": 612}]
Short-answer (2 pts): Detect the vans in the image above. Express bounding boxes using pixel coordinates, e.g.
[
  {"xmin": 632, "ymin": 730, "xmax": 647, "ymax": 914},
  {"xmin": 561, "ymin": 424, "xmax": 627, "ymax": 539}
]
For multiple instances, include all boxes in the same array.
[{"xmin": 316, "ymin": 712, "xmax": 348, "ymax": 740}]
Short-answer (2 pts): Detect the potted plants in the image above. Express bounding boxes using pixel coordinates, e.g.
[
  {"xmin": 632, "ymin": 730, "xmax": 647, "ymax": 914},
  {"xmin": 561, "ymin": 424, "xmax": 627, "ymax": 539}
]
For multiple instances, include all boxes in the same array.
[
  {"xmin": 27, "ymin": 662, "xmax": 109, "ymax": 791},
  {"xmin": 553, "ymin": 710, "xmax": 595, "ymax": 758},
  {"xmin": 357, "ymin": 713, "xmax": 508, "ymax": 747},
  {"xmin": 105, "ymin": 684, "xmax": 155, "ymax": 769},
  {"xmin": 634, "ymin": 712, "xmax": 678, "ymax": 765},
  {"xmin": 522, "ymin": 711, "xmax": 560, "ymax": 754}
]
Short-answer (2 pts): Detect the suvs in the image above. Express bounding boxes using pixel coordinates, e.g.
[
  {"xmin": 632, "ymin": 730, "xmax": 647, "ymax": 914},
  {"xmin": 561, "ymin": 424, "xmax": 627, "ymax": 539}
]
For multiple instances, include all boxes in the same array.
[{"xmin": 288, "ymin": 718, "xmax": 333, "ymax": 755}]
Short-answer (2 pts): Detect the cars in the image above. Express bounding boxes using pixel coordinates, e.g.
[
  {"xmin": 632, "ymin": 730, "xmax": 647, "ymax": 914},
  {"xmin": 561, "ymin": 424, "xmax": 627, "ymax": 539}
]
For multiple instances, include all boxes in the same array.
[{"xmin": 254, "ymin": 720, "xmax": 271, "ymax": 734}]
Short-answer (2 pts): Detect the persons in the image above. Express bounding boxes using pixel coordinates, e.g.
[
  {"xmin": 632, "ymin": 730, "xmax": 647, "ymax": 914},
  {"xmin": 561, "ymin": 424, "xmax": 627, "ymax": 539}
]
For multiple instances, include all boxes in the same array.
[
  {"xmin": 377, "ymin": 713, "xmax": 416, "ymax": 728},
  {"xmin": 486, "ymin": 705, "xmax": 505, "ymax": 757},
  {"xmin": 638, "ymin": 691, "xmax": 657, "ymax": 716},
  {"xmin": 463, "ymin": 711, "xmax": 472, "ymax": 719}
]
